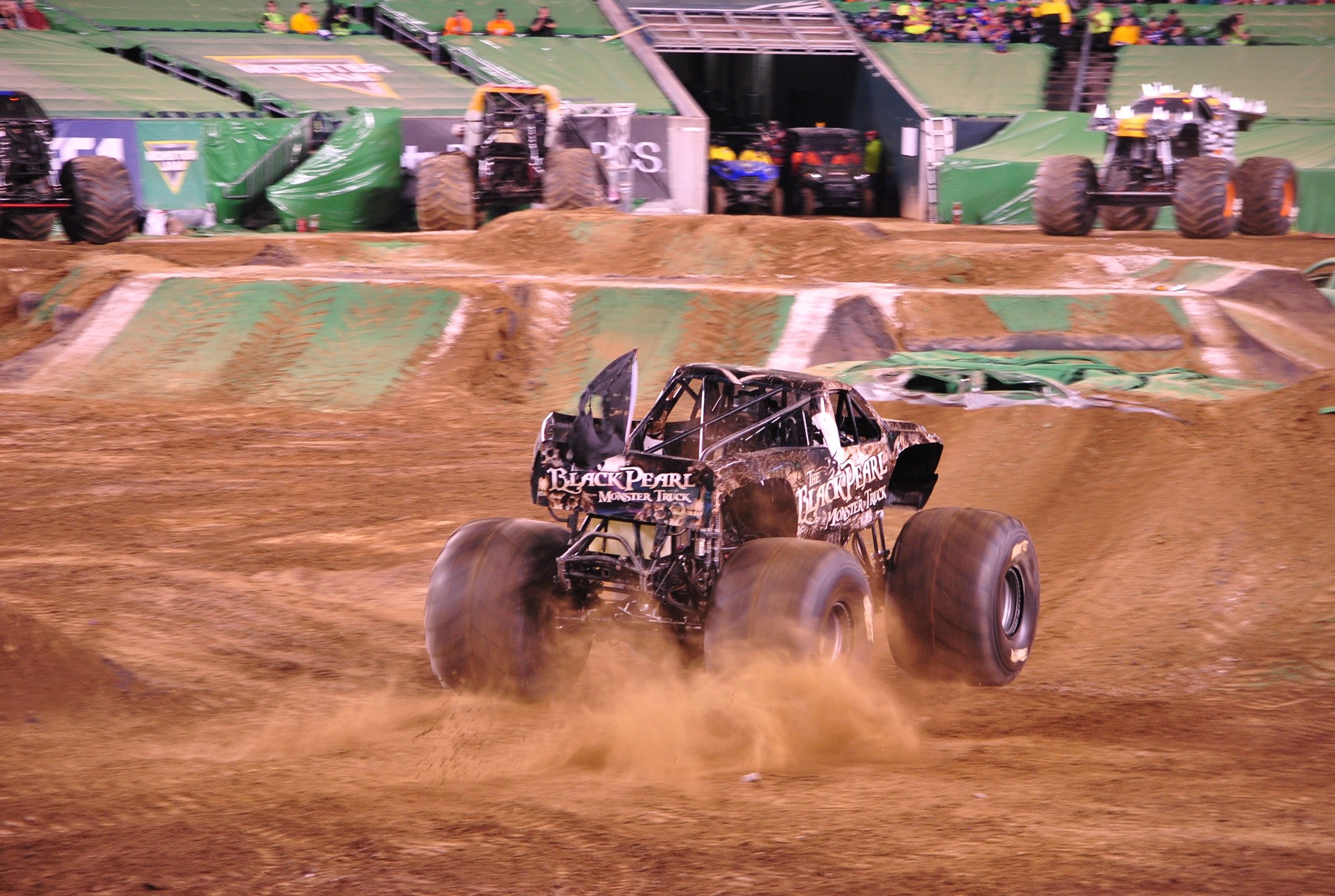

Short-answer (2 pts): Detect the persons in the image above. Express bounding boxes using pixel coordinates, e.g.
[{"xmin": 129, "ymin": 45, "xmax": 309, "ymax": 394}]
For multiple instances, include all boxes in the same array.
[
  {"xmin": 708, "ymin": 135, "xmax": 737, "ymax": 161},
  {"xmin": 841, "ymin": 0, "xmax": 1253, "ymax": 47},
  {"xmin": 790, "ymin": 138, "xmax": 823, "ymax": 172},
  {"xmin": 0, "ymin": 0, "xmax": 51, "ymax": 30},
  {"xmin": 258, "ymin": 0, "xmax": 354, "ymax": 39},
  {"xmin": 862, "ymin": 130, "xmax": 882, "ymax": 174},
  {"xmin": 757, "ymin": 121, "xmax": 785, "ymax": 164},
  {"xmin": 722, "ymin": 388, "xmax": 779, "ymax": 446},
  {"xmin": 831, "ymin": 141, "xmax": 862, "ymax": 165},
  {"xmin": 528, "ymin": 7, "xmax": 558, "ymax": 37},
  {"xmin": 737, "ymin": 141, "xmax": 773, "ymax": 164},
  {"xmin": 443, "ymin": 9, "xmax": 473, "ymax": 35},
  {"xmin": 486, "ymin": 8, "xmax": 516, "ymax": 36}
]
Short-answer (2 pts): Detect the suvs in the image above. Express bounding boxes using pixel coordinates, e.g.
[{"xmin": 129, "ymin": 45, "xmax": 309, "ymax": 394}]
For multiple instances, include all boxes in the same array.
[
  {"xmin": 0, "ymin": 91, "xmax": 138, "ymax": 244},
  {"xmin": 786, "ymin": 127, "xmax": 870, "ymax": 213},
  {"xmin": 416, "ymin": 82, "xmax": 597, "ymax": 230},
  {"xmin": 1035, "ymin": 82, "xmax": 1296, "ymax": 236},
  {"xmin": 708, "ymin": 143, "xmax": 786, "ymax": 217},
  {"xmin": 424, "ymin": 348, "xmax": 1040, "ymax": 702}
]
